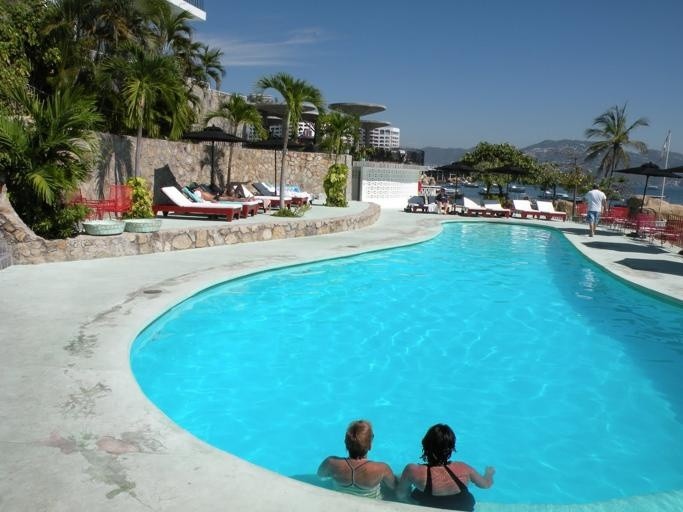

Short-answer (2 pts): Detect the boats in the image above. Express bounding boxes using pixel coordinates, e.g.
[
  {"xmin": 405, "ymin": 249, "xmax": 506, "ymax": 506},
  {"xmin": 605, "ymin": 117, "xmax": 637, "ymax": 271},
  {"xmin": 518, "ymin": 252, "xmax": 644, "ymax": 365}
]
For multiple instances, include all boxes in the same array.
[
  {"xmin": 646, "ymin": 185, "xmax": 658, "ymax": 190},
  {"xmin": 440, "ymin": 180, "xmax": 585, "ymax": 204}
]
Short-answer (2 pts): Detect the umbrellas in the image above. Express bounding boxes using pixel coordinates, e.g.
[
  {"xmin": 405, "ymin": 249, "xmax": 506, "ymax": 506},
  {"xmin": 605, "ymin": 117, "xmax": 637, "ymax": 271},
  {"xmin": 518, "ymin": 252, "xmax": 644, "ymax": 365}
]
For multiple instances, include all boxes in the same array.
[
  {"xmin": 434, "ymin": 160, "xmax": 483, "ymax": 216},
  {"xmin": 613, "ymin": 162, "xmax": 683, "ymax": 238},
  {"xmin": 484, "ymin": 163, "xmax": 535, "ymax": 211},
  {"xmin": 638, "ymin": 164, "xmax": 683, "ymax": 178},
  {"xmin": 181, "ymin": 124, "xmax": 249, "ymax": 184}
]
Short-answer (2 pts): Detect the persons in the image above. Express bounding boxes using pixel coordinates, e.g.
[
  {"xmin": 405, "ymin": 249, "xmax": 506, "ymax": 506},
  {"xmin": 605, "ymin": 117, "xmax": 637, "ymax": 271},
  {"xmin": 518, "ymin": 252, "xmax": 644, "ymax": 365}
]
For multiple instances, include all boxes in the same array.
[
  {"xmin": 317, "ymin": 419, "xmax": 400, "ymax": 499},
  {"xmin": 582, "ymin": 182, "xmax": 608, "ymax": 236},
  {"xmin": 434, "ymin": 187, "xmax": 450, "ymax": 215},
  {"xmin": 393, "ymin": 424, "xmax": 496, "ymax": 511},
  {"xmin": 190, "ymin": 182, "xmax": 251, "ymax": 201},
  {"xmin": 208, "ymin": 184, "xmax": 253, "ymax": 199}
]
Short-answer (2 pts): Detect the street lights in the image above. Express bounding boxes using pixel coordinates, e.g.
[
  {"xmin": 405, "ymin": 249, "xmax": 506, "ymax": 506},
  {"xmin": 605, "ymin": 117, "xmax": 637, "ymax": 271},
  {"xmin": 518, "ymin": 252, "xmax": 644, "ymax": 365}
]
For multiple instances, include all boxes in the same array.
[{"xmin": 656, "ymin": 127, "xmax": 676, "ymax": 215}]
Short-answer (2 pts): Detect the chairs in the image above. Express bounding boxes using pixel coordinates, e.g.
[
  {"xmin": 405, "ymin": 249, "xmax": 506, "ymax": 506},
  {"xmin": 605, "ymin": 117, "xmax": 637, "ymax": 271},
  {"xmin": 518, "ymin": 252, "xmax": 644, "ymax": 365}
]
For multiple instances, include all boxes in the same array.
[
  {"xmin": 404, "ymin": 191, "xmax": 682, "ymax": 251},
  {"xmin": 62, "ymin": 173, "xmax": 317, "ymax": 228}
]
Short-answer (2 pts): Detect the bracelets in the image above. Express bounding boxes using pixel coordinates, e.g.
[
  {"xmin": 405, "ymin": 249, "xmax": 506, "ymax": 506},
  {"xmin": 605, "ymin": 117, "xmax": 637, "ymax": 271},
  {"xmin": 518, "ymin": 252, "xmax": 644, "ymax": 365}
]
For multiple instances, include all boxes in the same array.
[{"xmin": 604, "ymin": 209, "xmax": 608, "ymax": 212}]
